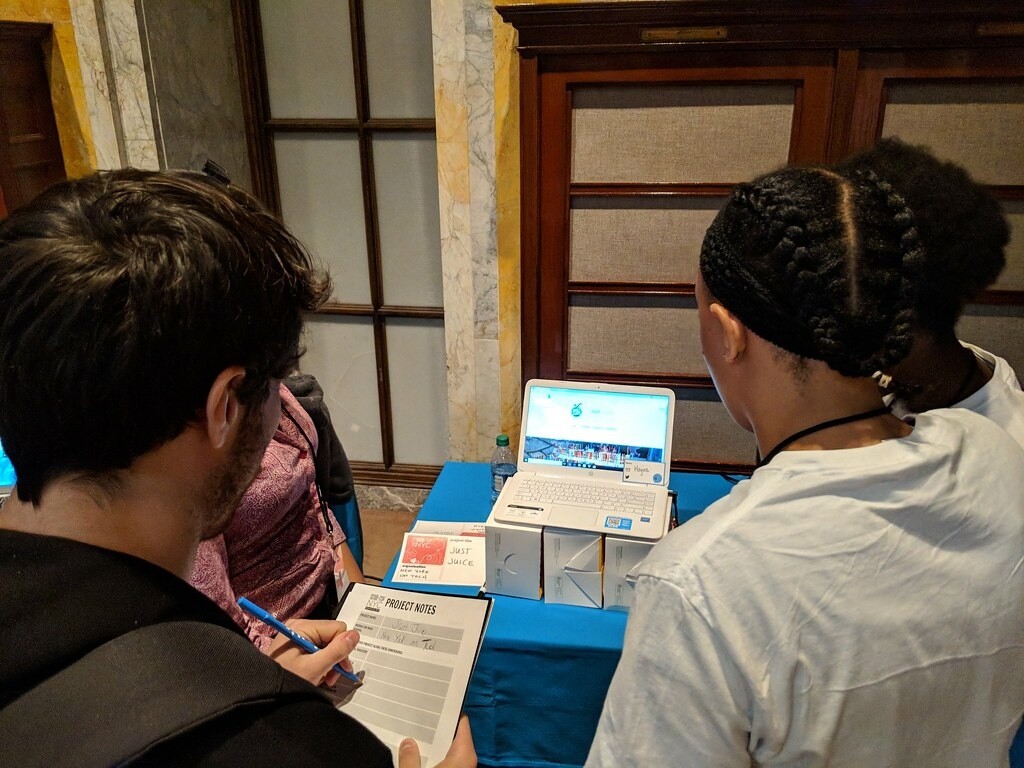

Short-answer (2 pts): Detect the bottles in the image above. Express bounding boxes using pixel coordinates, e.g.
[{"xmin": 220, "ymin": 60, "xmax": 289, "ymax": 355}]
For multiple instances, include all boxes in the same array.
[{"xmin": 491, "ymin": 435, "xmax": 517, "ymax": 502}]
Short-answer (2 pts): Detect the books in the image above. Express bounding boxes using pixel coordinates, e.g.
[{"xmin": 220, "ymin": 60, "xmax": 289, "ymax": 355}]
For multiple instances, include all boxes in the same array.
[{"xmin": 319, "ymin": 582, "xmax": 496, "ymax": 768}]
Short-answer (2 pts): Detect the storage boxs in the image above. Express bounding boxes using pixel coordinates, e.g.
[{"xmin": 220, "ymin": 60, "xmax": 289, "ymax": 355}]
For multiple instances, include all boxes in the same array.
[{"xmin": 484, "ymin": 478, "xmax": 672, "ymax": 611}]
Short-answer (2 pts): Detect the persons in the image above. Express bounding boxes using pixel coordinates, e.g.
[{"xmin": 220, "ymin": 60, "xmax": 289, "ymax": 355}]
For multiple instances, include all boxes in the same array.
[
  {"xmin": 0, "ymin": 167, "xmax": 478, "ymax": 768},
  {"xmin": 584, "ymin": 136, "xmax": 1024, "ymax": 768}
]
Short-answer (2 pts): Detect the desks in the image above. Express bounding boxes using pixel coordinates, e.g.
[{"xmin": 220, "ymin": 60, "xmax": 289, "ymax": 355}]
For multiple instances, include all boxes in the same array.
[{"xmin": 380, "ymin": 460, "xmax": 752, "ymax": 768}]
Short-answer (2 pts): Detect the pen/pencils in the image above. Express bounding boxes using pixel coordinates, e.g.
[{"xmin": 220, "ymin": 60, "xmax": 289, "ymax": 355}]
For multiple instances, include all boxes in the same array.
[{"xmin": 237, "ymin": 595, "xmax": 365, "ymax": 686}]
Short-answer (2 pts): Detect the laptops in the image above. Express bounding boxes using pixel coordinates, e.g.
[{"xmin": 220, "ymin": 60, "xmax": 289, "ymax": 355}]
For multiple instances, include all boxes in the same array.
[{"xmin": 493, "ymin": 378, "xmax": 676, "ymax": 541}]
